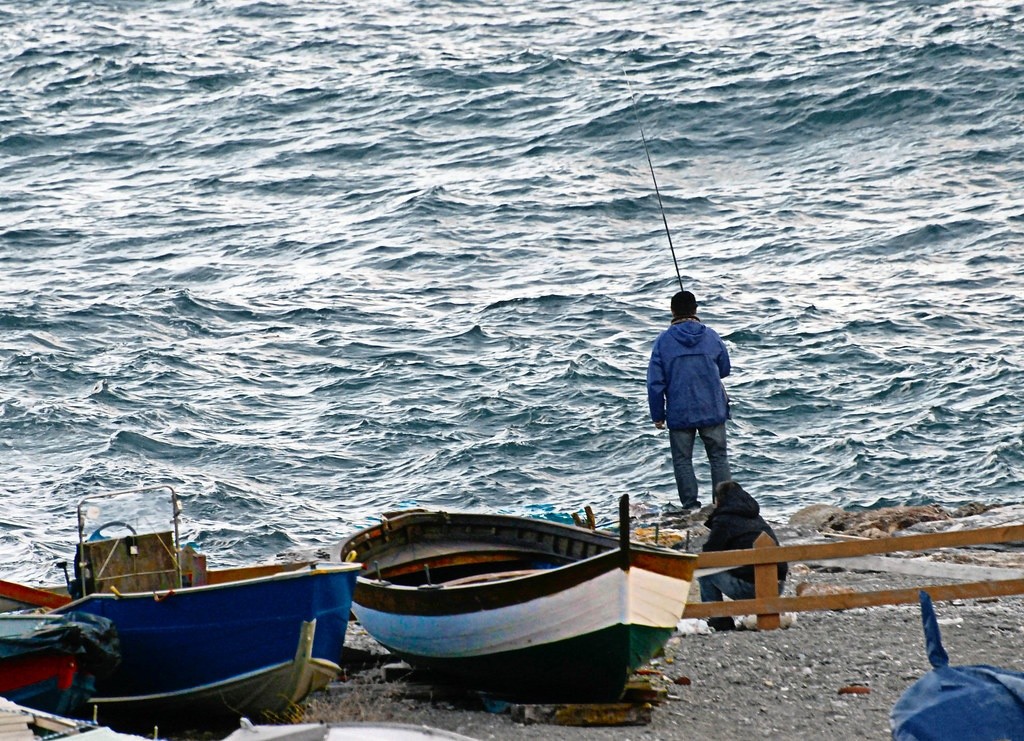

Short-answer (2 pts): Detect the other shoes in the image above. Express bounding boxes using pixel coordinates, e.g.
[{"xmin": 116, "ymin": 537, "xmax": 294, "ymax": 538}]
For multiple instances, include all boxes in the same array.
[
  {"xmin": 706, "ymin": 617, "xmax": 736, "ymax": 630},
  {"xmin": 686, "ymin": 503, "xmax": 701, "ymax": 514}
]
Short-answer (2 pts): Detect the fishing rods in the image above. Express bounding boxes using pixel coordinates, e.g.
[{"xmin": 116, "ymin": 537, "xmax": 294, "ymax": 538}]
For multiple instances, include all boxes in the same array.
[{"xmin": 620, "ymin": 63, "xmax": 685, "ymax": 292}]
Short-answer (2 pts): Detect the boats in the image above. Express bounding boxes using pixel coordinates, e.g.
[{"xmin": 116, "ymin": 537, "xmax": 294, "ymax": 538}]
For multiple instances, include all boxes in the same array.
[
  {"xmin": 334, "ymin": 502, "xmax": 701, "ymax": 704},
  {"xmin": 1, "ymin": 573, "xmax": 104, "ymax": 716},
  {"xmin": 33, "ymin": 485, "xmax": 365, "ymax": 726}
]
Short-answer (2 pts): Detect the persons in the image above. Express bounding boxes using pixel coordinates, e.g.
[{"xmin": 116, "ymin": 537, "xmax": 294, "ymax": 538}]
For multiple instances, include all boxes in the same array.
[
  {"xmin": 647, "ymin": 291, "xmax": 731, "ymax": 509},
  {"xmin": 699, "ymin": 480, "xmax": 788, "ymax": 631}
]
